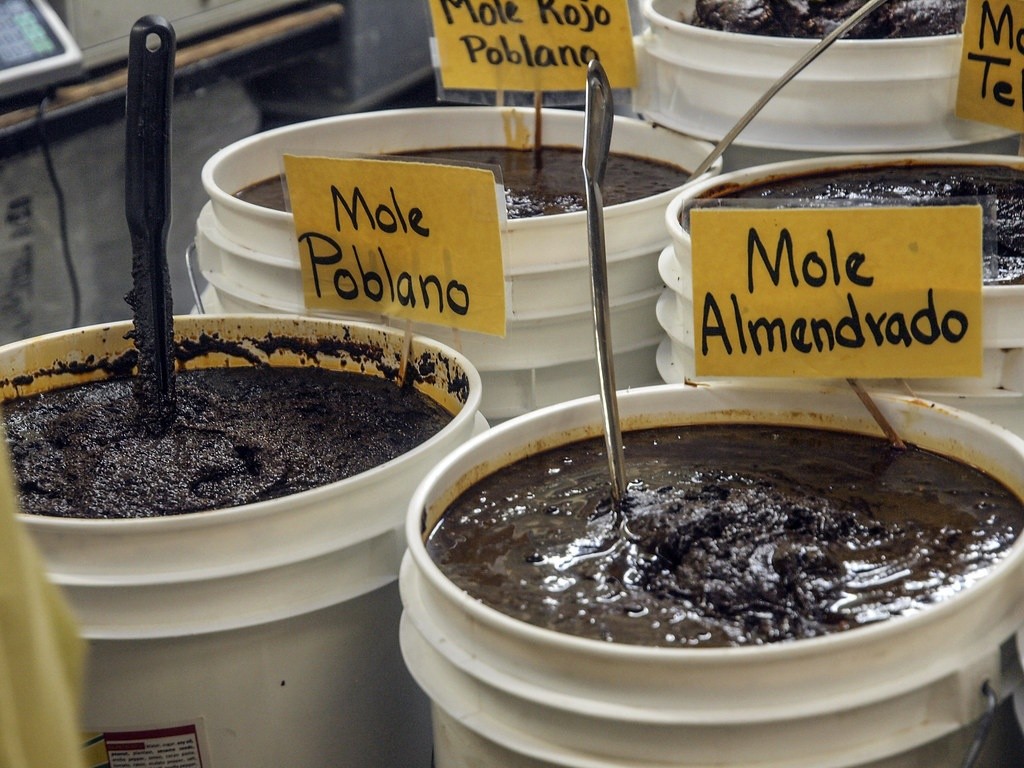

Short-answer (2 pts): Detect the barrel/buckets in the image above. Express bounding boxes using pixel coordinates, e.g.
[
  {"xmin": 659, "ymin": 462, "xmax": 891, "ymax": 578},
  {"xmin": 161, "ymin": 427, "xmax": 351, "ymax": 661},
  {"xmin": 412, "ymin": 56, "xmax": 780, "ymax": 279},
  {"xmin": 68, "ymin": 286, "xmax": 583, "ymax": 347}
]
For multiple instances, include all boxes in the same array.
[
  {"xmin": 185, "ymin": 105, "xmax": 724, "ymax": 423},
  {"xmin": 656, "ymin": 151, "xmax": 1024, "ymax": 438},
  {"xmin": 1, "ymin": 312, "xmax": 493, "ymax": 768},
  {"xmin": 631, "ymin": 0, "xmax": 1024, "ymax": 175},
  {"xmin": 396, "ymin": 380, "xmax": 1024, "ymax": 768}
]
